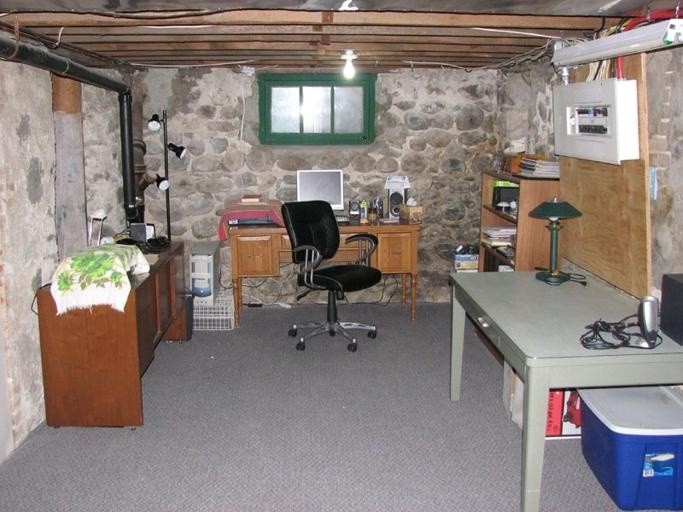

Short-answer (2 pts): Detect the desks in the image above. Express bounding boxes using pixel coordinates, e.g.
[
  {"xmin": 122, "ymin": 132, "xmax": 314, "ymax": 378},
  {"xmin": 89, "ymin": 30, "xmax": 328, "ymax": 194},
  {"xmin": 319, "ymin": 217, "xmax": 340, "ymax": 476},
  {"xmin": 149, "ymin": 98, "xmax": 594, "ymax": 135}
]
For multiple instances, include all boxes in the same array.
[
  {"xmin": 448, "ymin": 271, "xmax": 682, "ymax": 512},
  {"xmin": 227, "ymin": 224, "xmax": 418, "ymax": 329}
]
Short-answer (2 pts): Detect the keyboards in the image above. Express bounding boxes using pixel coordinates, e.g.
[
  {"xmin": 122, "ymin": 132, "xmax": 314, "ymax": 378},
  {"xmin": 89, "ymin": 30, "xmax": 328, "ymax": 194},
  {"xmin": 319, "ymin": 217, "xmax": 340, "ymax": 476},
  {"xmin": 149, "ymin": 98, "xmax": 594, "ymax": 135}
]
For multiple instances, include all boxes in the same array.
[{"xmin": 336, "ymin": 216, "xmax": 350, "ymax": 223}]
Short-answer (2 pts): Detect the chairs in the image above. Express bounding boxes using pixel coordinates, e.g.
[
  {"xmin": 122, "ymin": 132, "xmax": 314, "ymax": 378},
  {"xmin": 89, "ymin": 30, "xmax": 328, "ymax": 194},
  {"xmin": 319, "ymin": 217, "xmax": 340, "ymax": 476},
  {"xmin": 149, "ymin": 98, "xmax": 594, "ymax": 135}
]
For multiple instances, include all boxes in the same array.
[{"xmin": 282, "ymin": 201, "xmax": 382, "ymax": 353}]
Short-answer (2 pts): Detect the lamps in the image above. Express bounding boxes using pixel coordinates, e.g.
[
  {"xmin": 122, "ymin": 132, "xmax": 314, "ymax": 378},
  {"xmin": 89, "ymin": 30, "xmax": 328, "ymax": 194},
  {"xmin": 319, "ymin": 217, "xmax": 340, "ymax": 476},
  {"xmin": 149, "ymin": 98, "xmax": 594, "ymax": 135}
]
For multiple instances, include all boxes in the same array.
[
  {"xmin": 148, "ymin": 112, "xmax": 185, "ymax": 239},
  {"xmin": 526, "ymin": 194, "xmax": 586, "ymax": 287},
  {"xmin": 548, "ymin": 19, "xmax": 682, "ymax": 68},
  {"xmin": 340, "ymin": 49, "xmax": 358, "ymax": 81}
]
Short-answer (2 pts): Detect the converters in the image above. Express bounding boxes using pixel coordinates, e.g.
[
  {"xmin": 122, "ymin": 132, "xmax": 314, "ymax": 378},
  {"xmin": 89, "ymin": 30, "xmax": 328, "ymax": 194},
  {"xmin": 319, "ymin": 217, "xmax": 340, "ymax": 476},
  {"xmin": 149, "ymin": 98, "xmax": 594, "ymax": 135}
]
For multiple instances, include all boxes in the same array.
[
  {"xmin": 278, "ymin": 302, "xmax": 292, "ymax": 309},
  {"xmin": 247, "ymin": 301, "xmax": 263, "ymax": 307}
]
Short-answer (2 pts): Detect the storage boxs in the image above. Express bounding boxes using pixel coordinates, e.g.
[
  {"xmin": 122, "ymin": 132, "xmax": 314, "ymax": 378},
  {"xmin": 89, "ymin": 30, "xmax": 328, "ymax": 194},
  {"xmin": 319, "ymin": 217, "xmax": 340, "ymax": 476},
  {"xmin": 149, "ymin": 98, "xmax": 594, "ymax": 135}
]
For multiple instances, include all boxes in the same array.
[
  {"xmin": 502, "ymin": 357, "xmax": 581, "ymax": 440},
  {"xmin": 575, "ymin": 385, "xmax": 682, "ymax": 511}
]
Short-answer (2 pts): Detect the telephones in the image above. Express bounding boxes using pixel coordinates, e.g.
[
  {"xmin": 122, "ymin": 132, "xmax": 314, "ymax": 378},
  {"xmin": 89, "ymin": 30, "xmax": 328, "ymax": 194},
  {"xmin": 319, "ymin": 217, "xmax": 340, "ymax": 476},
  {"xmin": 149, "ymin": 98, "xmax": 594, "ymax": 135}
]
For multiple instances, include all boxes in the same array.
[{"xmin": 147, "ymin": 236, "xmax": 170, "ymax": 248}]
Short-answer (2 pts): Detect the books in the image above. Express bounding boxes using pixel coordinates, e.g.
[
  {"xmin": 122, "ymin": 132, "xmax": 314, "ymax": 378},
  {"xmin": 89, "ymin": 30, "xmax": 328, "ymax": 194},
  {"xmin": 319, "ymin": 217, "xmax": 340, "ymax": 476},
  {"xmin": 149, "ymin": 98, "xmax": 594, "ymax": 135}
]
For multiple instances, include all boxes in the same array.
[
  {"xmin": 239, "ymin": 194, "xmax": 264, "ymax": 203},
  {"xmin": 481, "ymin": 221, "xmax": 516, "ymax": 249},
  {"xmin": 514, "ymin": 155, "xmax": 564, "ymax": 181}
]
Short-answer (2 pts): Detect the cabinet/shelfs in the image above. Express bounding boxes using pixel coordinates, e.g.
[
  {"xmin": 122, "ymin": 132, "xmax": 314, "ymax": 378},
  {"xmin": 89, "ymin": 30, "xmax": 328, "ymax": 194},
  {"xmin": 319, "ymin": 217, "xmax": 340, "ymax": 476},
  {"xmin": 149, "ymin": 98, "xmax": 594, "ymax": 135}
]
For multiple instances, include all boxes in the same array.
[
  {"xmin": 167, "ymin": 238, "xmax": 190, "ymax": 340},
  {"xmin": 474, "ymin": 171, "xmax": 559, "ymax": 367},
  {"xmin": 36, "ymin": 270, "xmax": 156, "ymax": 431},
  {"xmin": 144, "ymin": 251, "xmax": 171, "ymax": 335}
]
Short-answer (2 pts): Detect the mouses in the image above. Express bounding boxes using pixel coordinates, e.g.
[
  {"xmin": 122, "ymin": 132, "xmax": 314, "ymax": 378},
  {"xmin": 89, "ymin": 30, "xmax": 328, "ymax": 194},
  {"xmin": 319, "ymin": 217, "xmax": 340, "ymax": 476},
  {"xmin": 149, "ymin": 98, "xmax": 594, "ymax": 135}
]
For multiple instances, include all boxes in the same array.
[{"xmin": 359, "ymin": 218, "xmax": 368, "ymax": 224}]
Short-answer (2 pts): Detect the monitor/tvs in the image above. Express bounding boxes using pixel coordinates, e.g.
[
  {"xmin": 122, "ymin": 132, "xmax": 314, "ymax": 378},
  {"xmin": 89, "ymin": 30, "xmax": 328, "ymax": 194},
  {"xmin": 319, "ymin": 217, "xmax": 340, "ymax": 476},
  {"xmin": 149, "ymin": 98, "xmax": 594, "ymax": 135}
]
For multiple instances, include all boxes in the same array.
[{"xmin": 296, "ymin": 169, "xmax": 345, "ymax": 212}]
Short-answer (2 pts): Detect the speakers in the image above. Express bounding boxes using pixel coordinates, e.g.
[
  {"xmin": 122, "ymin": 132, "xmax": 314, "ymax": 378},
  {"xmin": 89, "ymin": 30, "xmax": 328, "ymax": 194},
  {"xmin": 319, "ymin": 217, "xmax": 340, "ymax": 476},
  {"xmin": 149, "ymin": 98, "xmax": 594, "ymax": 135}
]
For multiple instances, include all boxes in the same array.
[{"xmin": 388, "ymin": 188, "xmax": 405, "ymax": 220}]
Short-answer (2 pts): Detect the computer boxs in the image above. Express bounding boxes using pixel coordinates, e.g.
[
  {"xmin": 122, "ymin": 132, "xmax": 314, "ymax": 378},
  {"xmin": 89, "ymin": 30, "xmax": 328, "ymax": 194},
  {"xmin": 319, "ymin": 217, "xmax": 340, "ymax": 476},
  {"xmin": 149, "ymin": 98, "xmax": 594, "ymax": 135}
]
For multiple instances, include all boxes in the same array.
[{"xmin": 188, "ymin": 241, "xmax": 221, "ymax": 305}]
